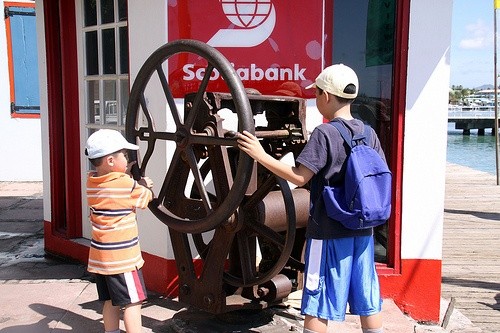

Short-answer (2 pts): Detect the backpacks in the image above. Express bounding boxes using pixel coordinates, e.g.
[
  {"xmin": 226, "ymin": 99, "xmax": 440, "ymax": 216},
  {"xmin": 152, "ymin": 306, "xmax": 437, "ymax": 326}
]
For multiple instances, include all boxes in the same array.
[{"xmin": 310, "ymin": 118, "xmax": 392, "ymax": 230}]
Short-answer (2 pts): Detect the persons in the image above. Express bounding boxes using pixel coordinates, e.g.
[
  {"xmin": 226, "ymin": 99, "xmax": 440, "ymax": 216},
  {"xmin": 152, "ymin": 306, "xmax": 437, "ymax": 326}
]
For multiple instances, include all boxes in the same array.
[
  {"xmin": 85, "ymin": 129, "xmax": 154, "ymax": 333},
  {"xmin": 237, "ymin": 64, "xmax": 388, "ymax": 333}
]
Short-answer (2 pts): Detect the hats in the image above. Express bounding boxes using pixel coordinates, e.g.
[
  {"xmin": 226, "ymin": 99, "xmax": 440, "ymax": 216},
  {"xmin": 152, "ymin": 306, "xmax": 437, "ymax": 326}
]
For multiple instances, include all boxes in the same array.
[
  {"xmin": 85, "ymin": 129, "xmax": 140, "ymax": 159},
  {"xmin": 305, "ymin": 64, "xmax": 359, "ymax": 98}
]
